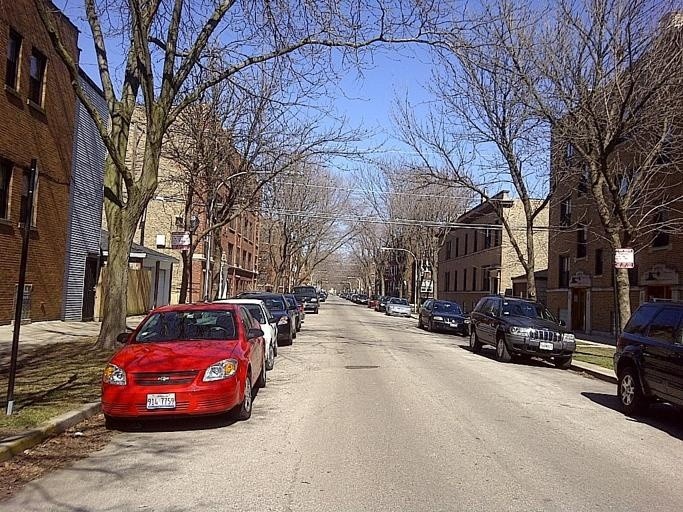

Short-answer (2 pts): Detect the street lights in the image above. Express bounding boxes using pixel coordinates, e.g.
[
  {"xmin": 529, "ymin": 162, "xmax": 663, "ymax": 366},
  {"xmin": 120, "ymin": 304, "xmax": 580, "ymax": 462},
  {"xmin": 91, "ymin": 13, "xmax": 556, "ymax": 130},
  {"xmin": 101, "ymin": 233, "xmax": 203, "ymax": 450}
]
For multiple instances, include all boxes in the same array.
[
  {"xmin": 202, "ymin": 167, "xmax": 306, "ymax": 302},
  {"xmin": 377, "ymin": 245, "xmax": 417, "ymax": 313}
]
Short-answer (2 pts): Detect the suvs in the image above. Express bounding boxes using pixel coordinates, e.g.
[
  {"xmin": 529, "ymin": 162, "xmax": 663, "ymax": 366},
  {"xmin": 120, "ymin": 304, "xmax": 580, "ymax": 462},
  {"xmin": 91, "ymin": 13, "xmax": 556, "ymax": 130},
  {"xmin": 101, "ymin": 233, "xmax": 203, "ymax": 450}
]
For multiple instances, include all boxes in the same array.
[
  {"xmin": 291, "ymin": 285, "xmax": 318, "ymax": 314},
  {"xmin": 469, "ymin": 292, "xmax": 577, "ymax": 370}
]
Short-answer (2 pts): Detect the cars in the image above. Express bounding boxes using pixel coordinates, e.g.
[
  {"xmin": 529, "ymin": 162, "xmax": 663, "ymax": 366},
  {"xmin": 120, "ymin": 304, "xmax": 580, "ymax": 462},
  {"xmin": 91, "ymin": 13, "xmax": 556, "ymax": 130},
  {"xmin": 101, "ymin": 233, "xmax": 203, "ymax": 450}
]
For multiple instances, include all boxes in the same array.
[
  {"xmin": 102, "ymin": 292, "xmax": 304, "ymax": 421},
  {"xmin": 345, "ymin": 292, "xmax": 368, "ymax": 304},
  {"xmin": 318, "ymin": 290, "xmax": 325, "ymax": 301},
  {"xmin": 419, "ymin": 298, "xmax": 470, "ymax": 335},
  {"xmin": 612, "ymin": 300, "xmax": 682, "ymax": 412},
  {"xmin": 369, "ymin": 295, "xmax": 411, "ymax": 318}
]
